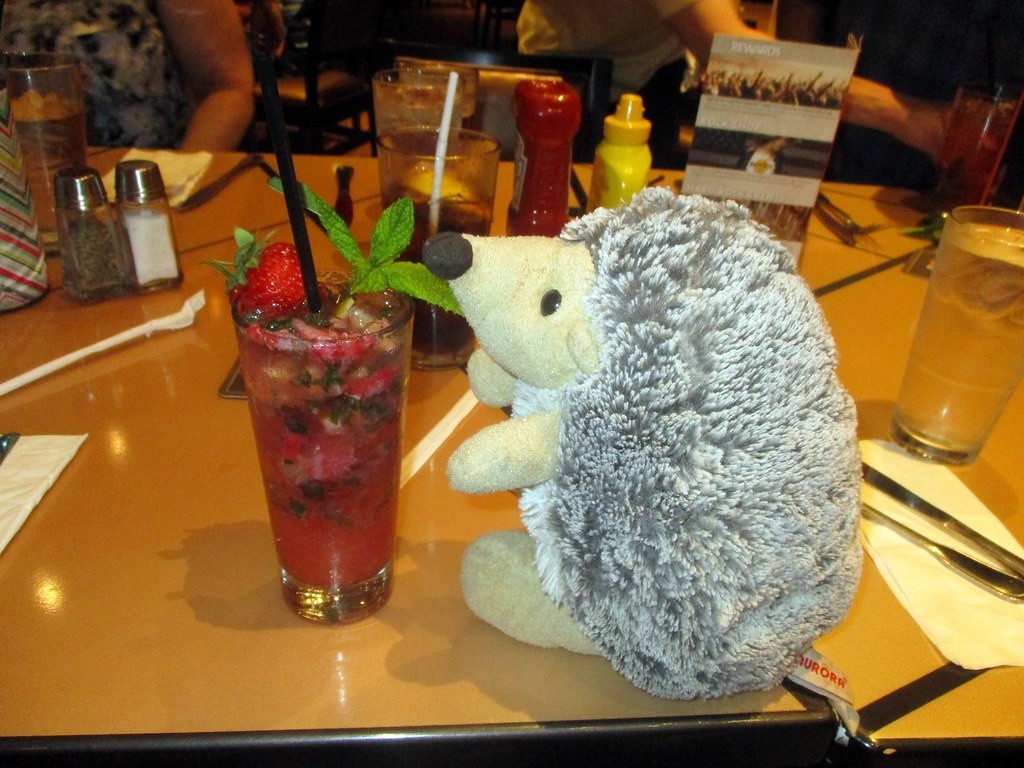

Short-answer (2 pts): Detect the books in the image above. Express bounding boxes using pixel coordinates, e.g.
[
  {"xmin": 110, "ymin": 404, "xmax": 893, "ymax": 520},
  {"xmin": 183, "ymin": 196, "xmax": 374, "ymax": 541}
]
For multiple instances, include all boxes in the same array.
[{"xmin": 682, "ymin": 30, "xmax": 866, "ymax": 261}]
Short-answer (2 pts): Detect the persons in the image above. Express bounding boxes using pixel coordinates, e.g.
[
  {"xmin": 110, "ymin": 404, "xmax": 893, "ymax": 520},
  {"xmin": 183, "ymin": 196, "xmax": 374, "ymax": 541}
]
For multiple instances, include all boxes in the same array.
[
  {"xmin": 515, "ymin": 2, "xmax": 989, "ymax": 169},
  {"xmin": 0, "ymin": 0, "xmax": 258, "ymax": 153}
]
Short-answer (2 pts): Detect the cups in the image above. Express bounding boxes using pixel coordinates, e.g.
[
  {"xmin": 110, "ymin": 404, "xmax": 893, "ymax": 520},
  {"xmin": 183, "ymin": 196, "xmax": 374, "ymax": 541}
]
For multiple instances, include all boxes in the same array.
[
  {"xmin": 932, "ymin": 78, "xmax": 1024, "ymax": 214},
  {"xmin": 231, "ymin": 279, "xmax": 416, "ymax": 624},
  {"xmin": 890, "ymin": 204, "xmax": 1024, "ymax": 466},
  {"xmin": 377, "ymin": 127, "xmax": 501, "ymax": 368},
  {"xmin": 373, "ymin": 61, "xmax": 482, "ymax": 133},
  {"xmin": 0, "ymin": 50, "xmax": 88, "ymax": 257}
]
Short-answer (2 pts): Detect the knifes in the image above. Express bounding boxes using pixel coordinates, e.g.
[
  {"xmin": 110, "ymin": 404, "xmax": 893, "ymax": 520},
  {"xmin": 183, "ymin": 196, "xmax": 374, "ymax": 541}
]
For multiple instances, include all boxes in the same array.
[
  {"xmin": 179, "ymin": 154, "xmax": 264, "ymax": 210},
  {"xmin": 861, "ymin": 462, "xmax": 1024, "ymax": 575},
  {"xmin": 813, "ymin": 206, "xmax": 855, "ymax": 247},
  {"xmin": 334, "ymin": 165, "xmax": 354, "ymax": 227}
]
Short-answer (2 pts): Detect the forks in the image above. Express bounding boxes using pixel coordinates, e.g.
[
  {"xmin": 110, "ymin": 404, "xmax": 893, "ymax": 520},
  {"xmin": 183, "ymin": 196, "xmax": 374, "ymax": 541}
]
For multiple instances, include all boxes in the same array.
[{"xmin": 817, "ymin": 191, "xmax": 888, "ymax": 235}]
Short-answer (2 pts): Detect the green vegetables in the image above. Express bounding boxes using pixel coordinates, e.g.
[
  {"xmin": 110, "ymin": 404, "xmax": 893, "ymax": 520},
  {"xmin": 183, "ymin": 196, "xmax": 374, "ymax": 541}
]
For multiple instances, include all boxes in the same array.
[{"xmin": 268, "ymin": 174, "xmax": 465, "ymax": 318}]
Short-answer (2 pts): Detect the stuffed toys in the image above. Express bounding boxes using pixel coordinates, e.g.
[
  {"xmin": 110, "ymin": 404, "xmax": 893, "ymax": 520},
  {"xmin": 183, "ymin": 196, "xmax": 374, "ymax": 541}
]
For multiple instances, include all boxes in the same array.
[{"xmin": 424, "ymin": 183, "xmax": 864, "ymax": 703}]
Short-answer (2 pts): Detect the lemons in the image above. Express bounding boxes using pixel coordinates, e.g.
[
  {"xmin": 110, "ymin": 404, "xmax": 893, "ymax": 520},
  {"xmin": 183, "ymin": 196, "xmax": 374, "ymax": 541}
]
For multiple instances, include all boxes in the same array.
[{"xmin": 411, "ymin": 172, "xmax": 474, "ymax": 202}]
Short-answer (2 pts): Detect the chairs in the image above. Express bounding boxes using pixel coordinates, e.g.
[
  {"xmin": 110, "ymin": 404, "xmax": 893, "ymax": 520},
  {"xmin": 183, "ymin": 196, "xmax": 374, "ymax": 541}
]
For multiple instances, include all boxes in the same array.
[{"xmin": 250, "ymin": 0, "xmax": 378, "ymax": 158}]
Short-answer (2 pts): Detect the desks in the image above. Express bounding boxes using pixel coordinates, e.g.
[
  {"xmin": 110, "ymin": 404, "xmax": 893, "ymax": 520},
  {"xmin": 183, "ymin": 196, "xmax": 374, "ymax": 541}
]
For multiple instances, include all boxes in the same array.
[
  {"xmin": 0, "ymin": 149, "xmax": 837, "ymax": 767},
  {"xmin": 569, "ymin": 161, "xmax": 1024, "ymax": 768}
]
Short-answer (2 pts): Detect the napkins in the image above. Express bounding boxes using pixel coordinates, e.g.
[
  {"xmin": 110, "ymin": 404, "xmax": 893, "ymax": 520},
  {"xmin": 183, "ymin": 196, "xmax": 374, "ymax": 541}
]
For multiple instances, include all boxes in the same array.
[
  {"xmin": 102, "ymin": 149, "xmax": 212, "ymax": 207},
  {"xmin": 858, "ymin": 439, "xmax": 1024, "ymax": 670},
  {"xmin": 0, "ymin": 433, "xmax": 88, "ymax": 554}
]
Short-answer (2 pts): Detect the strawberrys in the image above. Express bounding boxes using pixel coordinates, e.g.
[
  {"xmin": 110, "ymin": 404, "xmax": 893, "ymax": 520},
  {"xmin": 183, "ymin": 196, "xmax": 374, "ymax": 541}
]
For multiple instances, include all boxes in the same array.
[{"xmin": 199, "ymin": 226, "xmax": 309, "ymax": 323}]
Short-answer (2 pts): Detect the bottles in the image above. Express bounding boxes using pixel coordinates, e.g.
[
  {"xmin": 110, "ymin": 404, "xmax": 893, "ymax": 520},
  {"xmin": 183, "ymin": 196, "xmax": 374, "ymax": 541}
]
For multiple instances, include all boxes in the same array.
[
  {"xmin": 54, "ymin": 160, "xmax": 183, "ymax": 303},
  {"xmin": 506, "ymin": 77, "xmax": 652, "ymax": 239}
]
